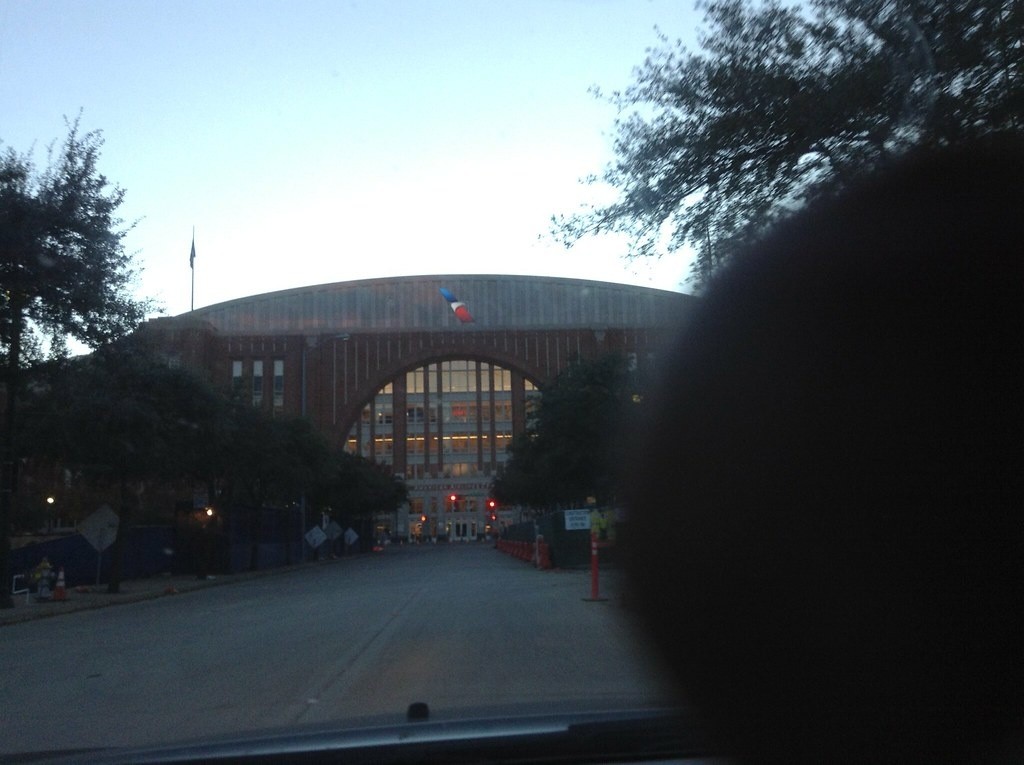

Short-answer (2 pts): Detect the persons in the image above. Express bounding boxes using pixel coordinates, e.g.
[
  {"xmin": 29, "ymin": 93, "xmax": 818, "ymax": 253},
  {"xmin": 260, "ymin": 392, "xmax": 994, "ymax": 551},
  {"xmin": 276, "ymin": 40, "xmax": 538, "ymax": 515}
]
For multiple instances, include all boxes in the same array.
[{"xmin": 382, "ymin": 529, "xmax": 391, "ymax": 554}]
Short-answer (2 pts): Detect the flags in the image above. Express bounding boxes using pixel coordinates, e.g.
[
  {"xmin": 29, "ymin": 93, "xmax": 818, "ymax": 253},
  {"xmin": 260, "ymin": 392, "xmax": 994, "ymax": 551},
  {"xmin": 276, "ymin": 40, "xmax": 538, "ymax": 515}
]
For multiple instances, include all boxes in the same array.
[{"xmin": 190, "ymin": 238, "xmax": 196, "ymax": 267}]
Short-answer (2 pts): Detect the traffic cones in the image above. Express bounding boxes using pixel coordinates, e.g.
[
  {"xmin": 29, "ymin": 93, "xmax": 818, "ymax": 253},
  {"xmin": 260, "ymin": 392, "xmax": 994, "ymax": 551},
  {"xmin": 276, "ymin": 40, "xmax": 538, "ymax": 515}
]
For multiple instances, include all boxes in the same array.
[{"xmin": 49, "ymin": 565, "xmax": 68, "ymax": 601}]
